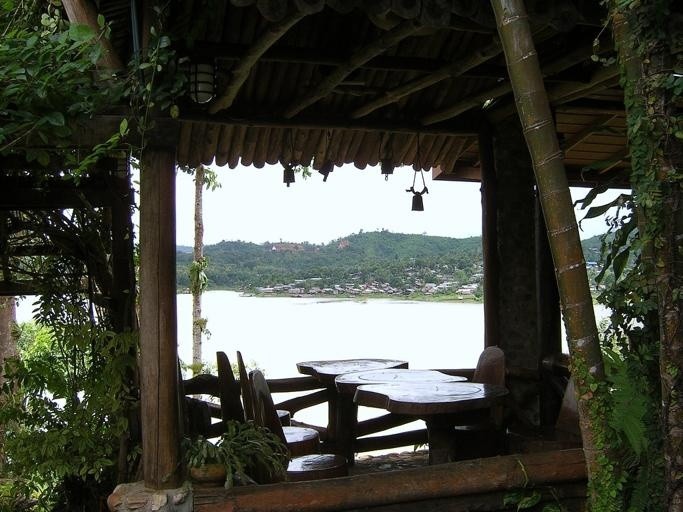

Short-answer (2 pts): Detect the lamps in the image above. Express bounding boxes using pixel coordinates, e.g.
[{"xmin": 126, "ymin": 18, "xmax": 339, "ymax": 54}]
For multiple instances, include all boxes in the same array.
[{"xmin": 185, "ymin": 59, "xmax": 219, "ymax": 106}]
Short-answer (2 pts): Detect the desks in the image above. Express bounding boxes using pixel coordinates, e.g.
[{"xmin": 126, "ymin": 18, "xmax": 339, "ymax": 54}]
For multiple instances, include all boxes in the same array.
[
  {"xmin": 295, "ymin": 358, "xmax": 410, "ymax": 460},
  {"xmin": 333, "ymin": 367, "xmax": 490, "ymax": 466}
]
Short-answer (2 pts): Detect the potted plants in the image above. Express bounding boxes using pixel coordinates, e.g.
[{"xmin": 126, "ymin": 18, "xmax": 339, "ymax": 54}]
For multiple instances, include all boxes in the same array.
[{"xmin": 180, "ymin": 419, "xmax": 293, "ymax": 490}]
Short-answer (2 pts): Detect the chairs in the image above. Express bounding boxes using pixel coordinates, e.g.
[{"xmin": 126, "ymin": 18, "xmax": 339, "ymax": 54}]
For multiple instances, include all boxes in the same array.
[
  {"xmin": 508, "ymin": 376, "xmax": 584, "ymax": 456},
  {"xmin": 217, "ymin": 351, "xmax": 293, "ymax": 426},
  {"xmin": 452, "ymin": 344, "xmax": 509, "ymax": 440},
  {"xmin": 235, "ymin": 350, "xmax": 322, "ymax": 455},
  {"xmin": 248, "ymin": 368, "xmax": 349, "ymax": 481}
]
